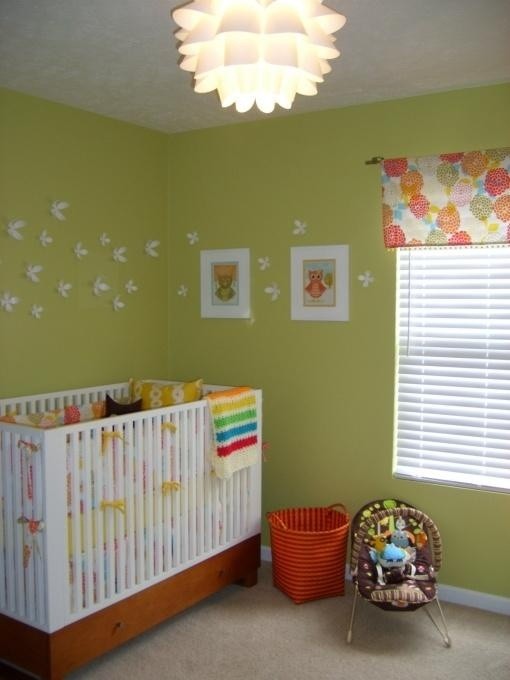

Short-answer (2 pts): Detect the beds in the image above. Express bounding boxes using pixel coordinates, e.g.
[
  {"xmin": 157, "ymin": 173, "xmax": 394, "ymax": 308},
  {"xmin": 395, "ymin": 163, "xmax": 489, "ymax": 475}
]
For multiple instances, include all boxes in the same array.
[{"xmin": 0, "ymin": 377, "xmax": 265, "ymax": 678}]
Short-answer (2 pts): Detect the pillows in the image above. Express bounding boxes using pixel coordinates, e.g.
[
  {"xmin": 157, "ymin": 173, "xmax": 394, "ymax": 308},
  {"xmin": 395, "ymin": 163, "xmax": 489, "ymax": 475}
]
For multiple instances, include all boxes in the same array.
[{"xmin": 128, "ymin": 378, "xmax": 203, "ymax": 412}]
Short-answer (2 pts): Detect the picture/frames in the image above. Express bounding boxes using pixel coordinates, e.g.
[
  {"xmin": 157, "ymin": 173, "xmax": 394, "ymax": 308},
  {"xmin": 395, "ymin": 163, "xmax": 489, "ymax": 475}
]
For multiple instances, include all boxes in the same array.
[{"xmin": 200, "ymin": 244, "xmax": 351, "ymax": 322}]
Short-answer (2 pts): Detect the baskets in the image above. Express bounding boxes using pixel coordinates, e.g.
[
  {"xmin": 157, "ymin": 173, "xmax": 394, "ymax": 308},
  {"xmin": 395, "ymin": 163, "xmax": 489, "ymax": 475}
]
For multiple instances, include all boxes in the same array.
[{"xmin": 266, "ymin": 503, "xmax": 350, "ymax": 604}]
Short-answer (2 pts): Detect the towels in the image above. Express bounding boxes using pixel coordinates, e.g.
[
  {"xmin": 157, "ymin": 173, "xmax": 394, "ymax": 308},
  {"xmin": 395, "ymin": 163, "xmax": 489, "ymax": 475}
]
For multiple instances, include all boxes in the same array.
[{"xmin": 203, "ymin": 386, "xmax": 258, "ymax": 480}]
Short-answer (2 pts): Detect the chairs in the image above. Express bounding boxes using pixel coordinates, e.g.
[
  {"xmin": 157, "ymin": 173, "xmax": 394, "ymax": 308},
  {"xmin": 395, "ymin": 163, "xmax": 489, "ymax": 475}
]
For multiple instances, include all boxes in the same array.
[{"xmin": 344, "ymin": 497, "xmax": 453, "ymax": 647}]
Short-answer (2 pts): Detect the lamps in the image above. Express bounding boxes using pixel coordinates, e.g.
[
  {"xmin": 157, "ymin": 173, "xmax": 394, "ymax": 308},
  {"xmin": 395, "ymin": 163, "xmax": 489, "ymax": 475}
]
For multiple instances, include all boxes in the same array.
[{"xmin": 169, "ymin": 1, "xmax": 347, "ymax": 116}]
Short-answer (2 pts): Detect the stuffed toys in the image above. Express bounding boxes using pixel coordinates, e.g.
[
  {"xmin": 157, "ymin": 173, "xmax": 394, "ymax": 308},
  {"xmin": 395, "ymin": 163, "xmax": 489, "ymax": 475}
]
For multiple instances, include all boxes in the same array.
[{"xmin": 371, "ymin": 529, "xmax": 424, "ymax": 585}]
